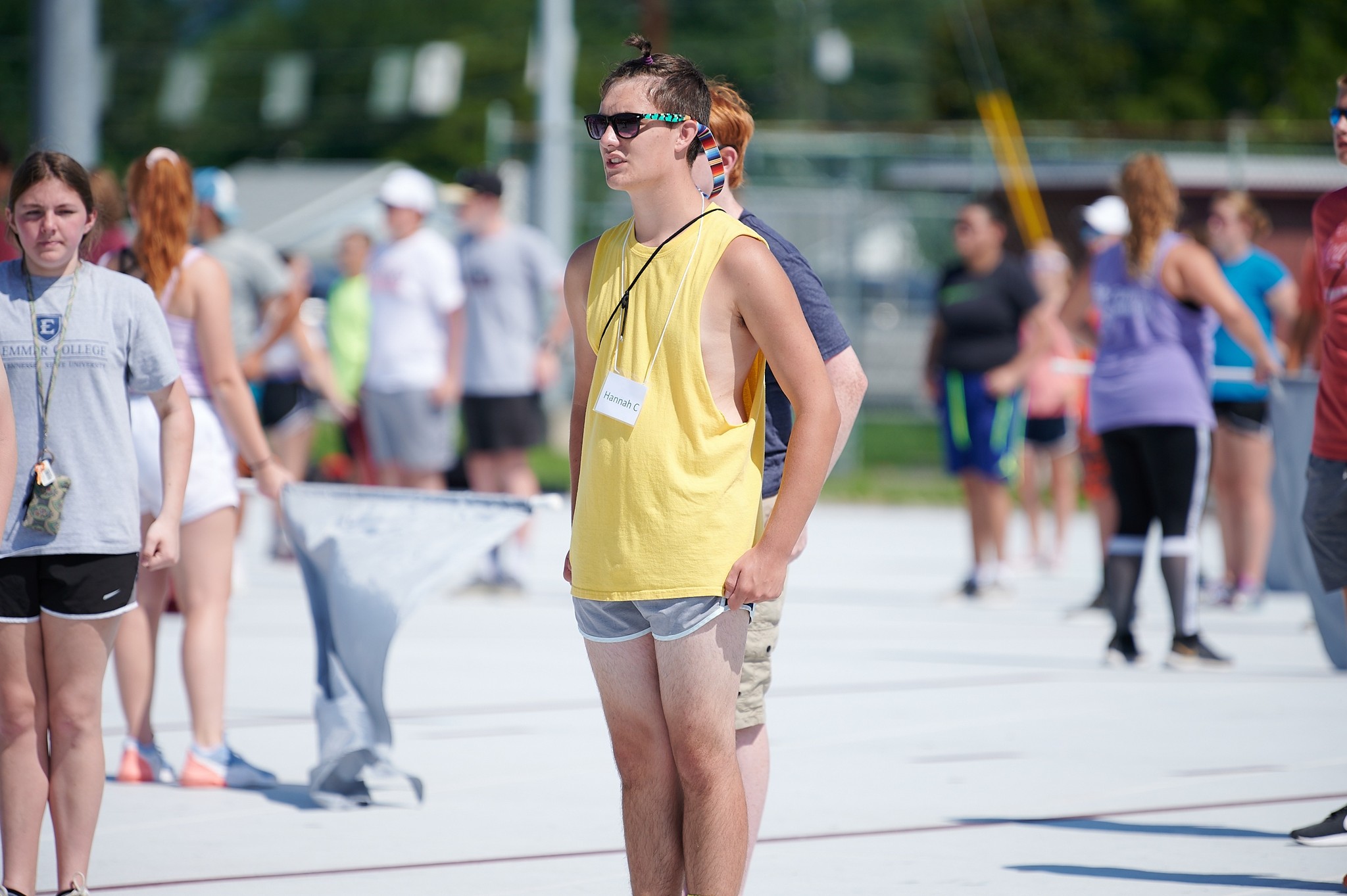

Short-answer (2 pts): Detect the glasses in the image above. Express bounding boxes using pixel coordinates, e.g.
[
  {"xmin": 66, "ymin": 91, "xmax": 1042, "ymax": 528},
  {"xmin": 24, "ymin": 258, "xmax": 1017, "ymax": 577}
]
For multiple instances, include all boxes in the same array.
[
  {"xmin": 583, "ymin": 113, "xmax": 691, "ymax": 140},
  {"xmin": 1329, "ymin": 108, "xmax": 1347, "ymax": 126},
  {"xmin": 1079, "ymin": 227, "xmax": 1099, "ymax": 239},
  {"xmin": 947, "ymin": 218, "xmax": 972, "ymax": 234}
]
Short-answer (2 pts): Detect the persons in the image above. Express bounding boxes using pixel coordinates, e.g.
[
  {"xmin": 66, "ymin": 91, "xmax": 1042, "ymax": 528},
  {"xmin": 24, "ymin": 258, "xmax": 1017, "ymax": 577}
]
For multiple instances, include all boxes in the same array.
[
  {"xmin": 0, "ymin": 145, "xmax": 571, "ymax": 896},
  {"xmin": 1290, "ymin": 71, "xmax": 1347, "ymax": 891},
  {"xmin": 929, "ymin": 146, "xmax": 1300, "ymax": 668},
  {"xmin": 562, "ymin": 31, "xmax": 842, "ymax": 896},
  {"xmin": 689, "ymin": 74, "xmax": 869, "ymax": 896}
]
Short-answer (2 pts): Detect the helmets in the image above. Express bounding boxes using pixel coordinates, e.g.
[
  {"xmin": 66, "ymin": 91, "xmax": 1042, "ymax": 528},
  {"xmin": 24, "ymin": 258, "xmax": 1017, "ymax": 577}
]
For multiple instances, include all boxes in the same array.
[{"xmin": 375, "ymin": 168, "xmax": 437, "ymax": 217}]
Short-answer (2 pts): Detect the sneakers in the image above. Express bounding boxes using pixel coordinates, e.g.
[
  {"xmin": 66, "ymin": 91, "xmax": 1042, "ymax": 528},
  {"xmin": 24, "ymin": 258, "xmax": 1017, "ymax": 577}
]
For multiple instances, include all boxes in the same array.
[
  {"xmin": 1291, "ymin": 805, "xmax": 1347, "ymax": 888},
  {"xmin": 117, "ymin": 741, "xmax": 176, "ymax": 784},
  {"xmin": 181, "ymin": 745, "xmax": 276, "ymax": 789}
]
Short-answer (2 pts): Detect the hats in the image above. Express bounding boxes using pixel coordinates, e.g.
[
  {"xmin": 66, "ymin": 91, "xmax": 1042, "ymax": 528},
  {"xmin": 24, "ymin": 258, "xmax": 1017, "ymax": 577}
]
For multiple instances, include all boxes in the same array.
[
  {"xmin": 189, "ymin": 167, "xmax": 249, "ymax": 227},
  {"xmin": 439, "ymin": 167, "xmax": 502, "ymax": 203},
  {"xmin": 1078, "ymin": 195, "xmax": 1132, "ymax": 236}
]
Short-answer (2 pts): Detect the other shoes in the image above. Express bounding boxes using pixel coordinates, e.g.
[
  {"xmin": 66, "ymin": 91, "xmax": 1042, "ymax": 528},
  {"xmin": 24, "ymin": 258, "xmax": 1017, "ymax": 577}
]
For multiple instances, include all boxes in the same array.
[
  {"xmin": 1108, "ymin": 630, "xmax": 1141, "ymax": 666},
  {"xmin": 0, "ymin": 871, "xmax": 91, "ymax": 896},
  {"xmin": 1167, "ymin": 630, "xmax": 1234, "ymax": 671}
]
